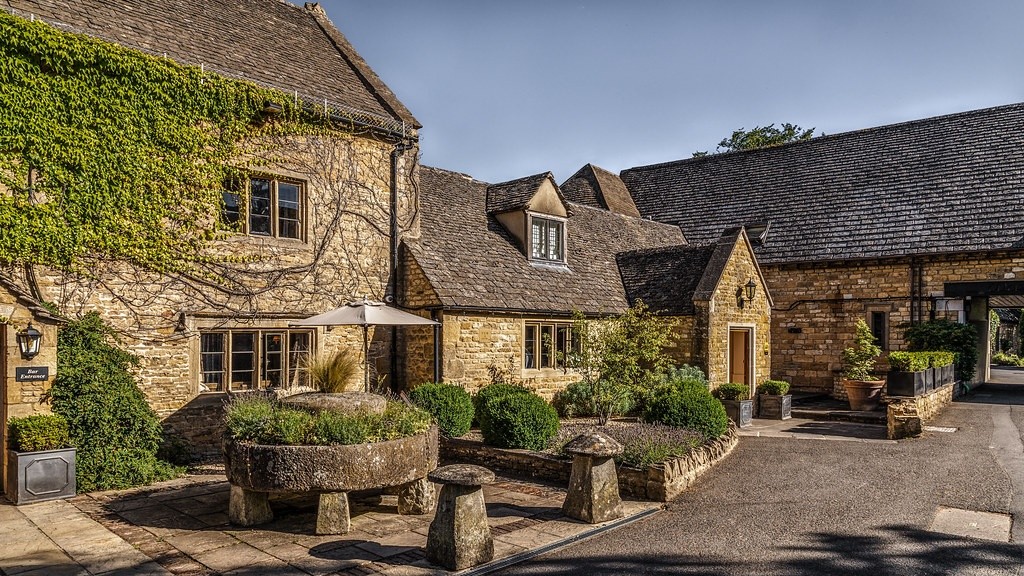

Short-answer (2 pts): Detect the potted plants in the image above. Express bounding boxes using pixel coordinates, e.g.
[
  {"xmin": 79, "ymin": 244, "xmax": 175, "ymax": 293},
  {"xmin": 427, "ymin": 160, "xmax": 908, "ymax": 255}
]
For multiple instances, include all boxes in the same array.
[
  {"xmin": 910, "ymin": 352, "xmax": 933, "ymax": 392},
  {"xmin": 887, "ymin": 351, "xmax": 925, "ymax": 396},
  {"xmin": 838, "ymin": 318, "xmax": 885, "ymax": 411},
  {"xmin": 717, "ymin": 383, "xmax": 753, "ymax": 429},
  {"xmin": 946, "ymin": 352, "xmax": 955, "ymax": 383},
  {"xmin": 929, "ymin": 351, "xmax": 941, "ymax": 390},
  {"xmin": 940, "ymin": 352, "xmax": 949, "ymax": 386},
  {"xmin": 6, "ymin": 413, "xmax": 76, "ymax": 506},
  {"xmin": 759, "ymin": 380, "xmax": 793, "ymax": 421}
]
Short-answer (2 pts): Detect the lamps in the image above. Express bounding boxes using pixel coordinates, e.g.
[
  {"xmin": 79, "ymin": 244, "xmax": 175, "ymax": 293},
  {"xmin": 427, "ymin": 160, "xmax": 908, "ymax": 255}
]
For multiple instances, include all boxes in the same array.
[
  {"xmin": 741, "ymin": 277, "xmax": 757, "ymax": 309},
  {"xmin": 16, "ymin": 321, "xmax": 43, "ymax": 361}
]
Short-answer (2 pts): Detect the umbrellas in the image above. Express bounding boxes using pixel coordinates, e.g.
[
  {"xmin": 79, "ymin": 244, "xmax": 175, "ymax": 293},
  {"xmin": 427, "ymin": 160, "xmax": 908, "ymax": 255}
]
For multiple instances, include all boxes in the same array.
[{"xmin": 288, "ymin": 294, "xmax": 442, "ymax": 394}]
